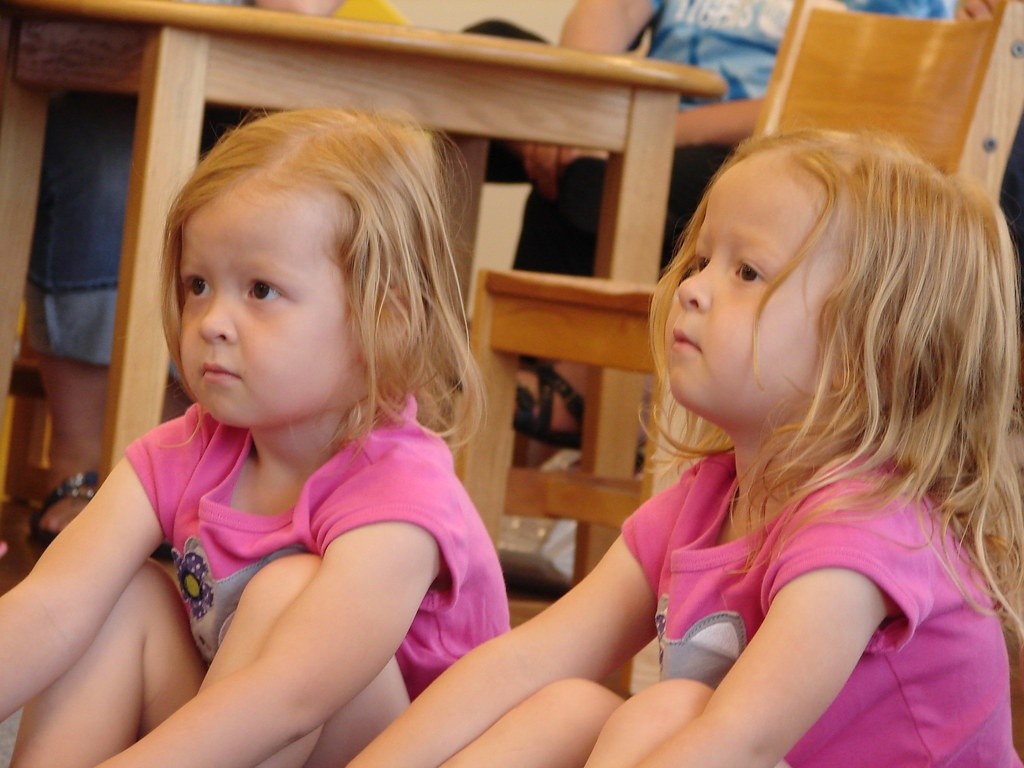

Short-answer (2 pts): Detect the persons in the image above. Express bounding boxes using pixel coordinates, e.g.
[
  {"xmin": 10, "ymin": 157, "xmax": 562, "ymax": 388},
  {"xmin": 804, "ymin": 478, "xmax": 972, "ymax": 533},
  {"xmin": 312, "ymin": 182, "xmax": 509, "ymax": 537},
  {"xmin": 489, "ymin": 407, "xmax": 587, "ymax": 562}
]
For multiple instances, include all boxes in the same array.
[
  {"xmin": 25, "ymin": 1, "xmax": 348, "ymax": 546},
  {"xmin": 0, "ymin": 111, "xmax": 511, "ymax": 767},
  {"xmin": 342, "ymin": 128, "xmax": 1023, "ymax": 768},
  {"xmin": 513, "ymin": 1, "xmax": 993, "ymax": 451}
]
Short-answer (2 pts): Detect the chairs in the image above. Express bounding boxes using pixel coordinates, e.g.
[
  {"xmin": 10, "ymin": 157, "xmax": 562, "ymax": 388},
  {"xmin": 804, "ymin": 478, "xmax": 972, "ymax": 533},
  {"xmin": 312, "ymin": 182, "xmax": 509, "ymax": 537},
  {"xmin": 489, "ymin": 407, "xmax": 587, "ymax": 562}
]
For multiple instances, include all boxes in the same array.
[{"xmin": 454, "ymin": 1, "xmax": 1023, "ymax": 562}]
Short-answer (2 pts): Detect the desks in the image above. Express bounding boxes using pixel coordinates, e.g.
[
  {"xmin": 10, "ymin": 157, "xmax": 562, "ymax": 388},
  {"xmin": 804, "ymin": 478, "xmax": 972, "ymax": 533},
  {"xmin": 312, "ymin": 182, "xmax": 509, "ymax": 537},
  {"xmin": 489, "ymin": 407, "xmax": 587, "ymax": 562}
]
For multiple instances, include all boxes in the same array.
[{"xmin": 0, "ymin": 0, "xmax": 728, "ymax": 699}]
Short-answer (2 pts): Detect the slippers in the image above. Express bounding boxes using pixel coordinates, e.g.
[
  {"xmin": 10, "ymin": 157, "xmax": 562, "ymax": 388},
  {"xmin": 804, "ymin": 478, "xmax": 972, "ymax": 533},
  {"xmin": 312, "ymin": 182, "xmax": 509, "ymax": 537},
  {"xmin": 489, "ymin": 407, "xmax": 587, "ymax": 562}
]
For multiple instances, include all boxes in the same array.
[
  {"xmin": 30, "ymin": 471, "xmax": 100, "ymax": 543},
  {"xmin": 512, "ymin": 354, "xmax": 586, "ymax": 449}
]
